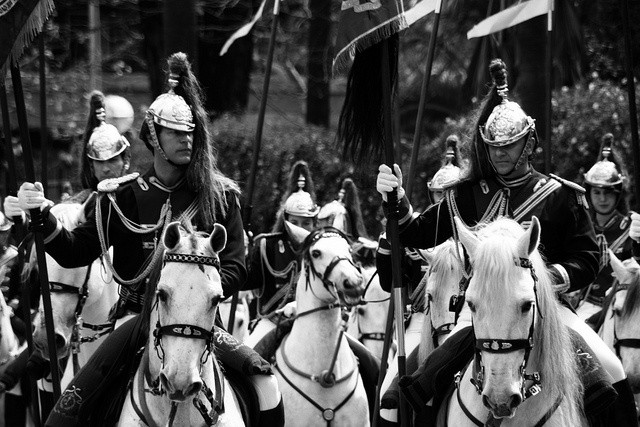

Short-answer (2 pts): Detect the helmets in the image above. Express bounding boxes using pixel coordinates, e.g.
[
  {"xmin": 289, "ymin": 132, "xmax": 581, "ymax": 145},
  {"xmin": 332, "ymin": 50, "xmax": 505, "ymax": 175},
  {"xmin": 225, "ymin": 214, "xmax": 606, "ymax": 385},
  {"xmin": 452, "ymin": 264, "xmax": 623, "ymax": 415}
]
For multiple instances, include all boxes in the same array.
[
  {"xmin": 471, "ymin": 58, "xmax": 539, "ymax": 178},
  {"xmin": 80, "ymin": 90, "xmax": 131, "ymax": 189},
  {"xmin": 427, "ymin": 135, "xmax": 471, "ymax": 204},
  {"xmin": 139, "ymin": 52, "xmax": 241, "ymax": 225},
  {"xmin": 582, "ymin": 133, "xmax": 630, "ymax": 216},
  {"xmin": 317, "ymin": 178, "xmax": 369, "ymax": 241}
]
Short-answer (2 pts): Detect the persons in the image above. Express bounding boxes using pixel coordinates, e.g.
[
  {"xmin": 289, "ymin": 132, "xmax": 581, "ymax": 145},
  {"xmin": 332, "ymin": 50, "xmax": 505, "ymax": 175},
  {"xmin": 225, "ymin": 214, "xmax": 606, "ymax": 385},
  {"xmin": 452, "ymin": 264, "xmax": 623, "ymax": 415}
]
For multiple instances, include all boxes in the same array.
[
  {"xmin": 17, "ymin": 52, "xmax": 283, "ymax": 427},
  {"xmin": 379, "ymin": 132, "xmax": 471, "ymax": 421},
  {"xmin": 316, "ymin": 176, "xmax": 376, "ymax": 268},
  {"xmin": 233, "ymin": 160, "xmax": 380, "ymax": 427},
  {"xmin": 376, "ymin": 58, "xmax": 640, "ymax": 427},
  {"xmin": 574, "ymin": 132, "xmax": 639, "ymax": 334},
  {"xmin": 3, "ymin": 88, "xmax": 131, "ymax": 231},
  {"xmin": 0, "ymin": 213, "xmax": 29, "ymax": 426}
]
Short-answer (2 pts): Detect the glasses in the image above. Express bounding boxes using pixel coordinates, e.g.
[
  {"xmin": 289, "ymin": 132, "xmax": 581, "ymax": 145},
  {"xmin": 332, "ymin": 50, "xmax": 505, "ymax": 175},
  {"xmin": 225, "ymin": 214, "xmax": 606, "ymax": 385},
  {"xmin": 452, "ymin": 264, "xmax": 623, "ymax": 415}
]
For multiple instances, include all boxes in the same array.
[{"xmin": 272, "ymin": 161, "xmax": 320, "ymax": 245}]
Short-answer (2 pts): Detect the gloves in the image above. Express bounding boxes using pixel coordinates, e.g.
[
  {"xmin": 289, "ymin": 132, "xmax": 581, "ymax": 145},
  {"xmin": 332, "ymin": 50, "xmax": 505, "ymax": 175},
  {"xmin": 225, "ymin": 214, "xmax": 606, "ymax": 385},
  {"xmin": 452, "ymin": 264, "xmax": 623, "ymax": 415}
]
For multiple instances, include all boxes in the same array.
[
  {"xmin": 628, "ymin": 213, "xmax": 639, "ymax": 242},
  {"xmin": 376, "ymin": 164, "xmax": 405, "ymax": 202},
  {"xmin": 17, "ymin": 181, "xmax": 44, "ymax": 214},
  {"xmin": 3, "ymin": 195, "xmax": 26, "ymax": 223}
]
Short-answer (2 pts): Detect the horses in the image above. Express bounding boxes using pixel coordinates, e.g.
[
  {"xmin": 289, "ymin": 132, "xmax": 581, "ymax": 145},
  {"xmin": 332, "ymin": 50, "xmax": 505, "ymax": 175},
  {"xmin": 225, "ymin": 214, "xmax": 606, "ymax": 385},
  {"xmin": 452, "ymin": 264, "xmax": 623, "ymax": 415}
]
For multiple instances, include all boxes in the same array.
[
  {"xmin": 119, "ymin": 219, "xmax": 245, "ymax": 426},
  {"xmin": 417, "ymin": 236, "xmax": 472, "ymax": 368},
  {"xmin": 445, "ymin": 216, "xmax": 588, "ymax": 427},
  {"xmin": 29, "ymin": 181, "xmax": 119, "ymax": 395},
  {"xmin": 245, "ymin": 219, "xmax": 369, "ymax": 426},
  {"xmin": 218, "ymin": 289, "xmax": 252, "ymax": 347},
  {"xmin": 346, "ymin": 265, "xmax": 402, "ymax": 373},
  {"xmin": 602, "ymin": 248, "xmax": 640, "ymax": 393}
]
